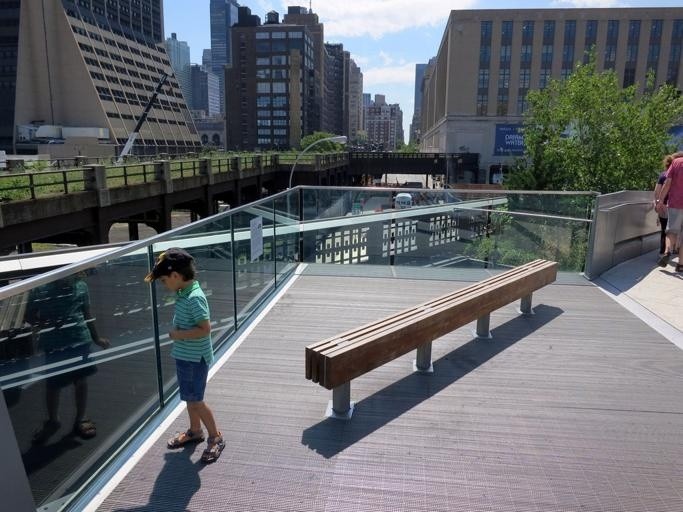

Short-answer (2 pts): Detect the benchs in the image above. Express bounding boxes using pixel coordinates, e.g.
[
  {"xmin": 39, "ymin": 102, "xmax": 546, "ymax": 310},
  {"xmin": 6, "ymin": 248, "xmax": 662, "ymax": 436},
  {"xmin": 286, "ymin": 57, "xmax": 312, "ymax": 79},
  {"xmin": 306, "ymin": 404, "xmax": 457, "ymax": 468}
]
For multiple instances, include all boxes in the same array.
[{"xmin": 305, "ymin": 257, "xmax": 558, "ymax": 420}]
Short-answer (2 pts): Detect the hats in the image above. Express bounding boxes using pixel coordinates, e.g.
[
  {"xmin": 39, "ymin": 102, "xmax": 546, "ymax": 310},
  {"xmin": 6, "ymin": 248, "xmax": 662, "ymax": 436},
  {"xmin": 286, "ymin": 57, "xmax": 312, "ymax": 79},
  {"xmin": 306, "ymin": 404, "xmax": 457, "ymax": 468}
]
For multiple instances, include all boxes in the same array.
[{"xmin": 144, "ymin": 247, "xmax": 194, "ymax": 282}]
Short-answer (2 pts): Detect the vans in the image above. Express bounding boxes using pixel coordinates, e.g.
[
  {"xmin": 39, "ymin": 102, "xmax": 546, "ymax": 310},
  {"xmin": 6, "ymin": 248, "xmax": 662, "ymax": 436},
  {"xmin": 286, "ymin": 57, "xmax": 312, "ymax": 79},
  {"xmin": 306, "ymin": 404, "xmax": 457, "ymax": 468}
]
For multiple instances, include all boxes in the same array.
[{"xmin": 393, "ymin": 193, "xmax": 412, "ymax": 208}]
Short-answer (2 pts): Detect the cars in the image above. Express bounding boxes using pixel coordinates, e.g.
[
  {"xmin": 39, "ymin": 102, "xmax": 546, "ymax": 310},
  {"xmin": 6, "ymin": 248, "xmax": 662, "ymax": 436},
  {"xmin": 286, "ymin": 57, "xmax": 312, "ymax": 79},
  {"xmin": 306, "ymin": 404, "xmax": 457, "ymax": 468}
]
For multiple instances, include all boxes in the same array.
[{"xmin": 400, "ymin": 182, "xmax": 422, "ymax": 188}]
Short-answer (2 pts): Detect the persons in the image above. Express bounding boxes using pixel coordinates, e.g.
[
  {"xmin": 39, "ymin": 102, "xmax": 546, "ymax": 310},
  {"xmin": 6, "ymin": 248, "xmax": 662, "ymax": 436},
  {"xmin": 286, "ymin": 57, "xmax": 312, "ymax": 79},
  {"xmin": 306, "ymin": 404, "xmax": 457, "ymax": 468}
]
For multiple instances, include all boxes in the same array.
[
  {"xmin": 653, "ymin": 152, "xmax": 683, "ymax": 259},
  {"xmin": 21, "ymin": 268, "xmax": 111, "ymax": 443},
  {"xmin": 654, "ymin": 157, "xmax": 683, "ymax": 273},
  {"xmin": 144, "ymin": 246, "xmax": 226, "ymax": 464}
]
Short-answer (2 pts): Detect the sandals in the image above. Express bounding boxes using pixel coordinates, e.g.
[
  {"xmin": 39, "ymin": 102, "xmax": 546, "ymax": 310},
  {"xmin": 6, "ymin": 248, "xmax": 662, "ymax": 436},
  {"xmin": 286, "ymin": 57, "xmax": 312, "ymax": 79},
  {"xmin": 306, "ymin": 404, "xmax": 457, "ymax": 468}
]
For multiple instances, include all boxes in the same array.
[
  {"xmin": 656, "ymin": 247, "xmax": 683, "ymax": 272},
  {"xmin": 200, "ymin": 431, "xmax": 226, "ymax": 465},
  {"xmin": 167, "ymin": 427, "xmax": 206, "ymax": 448}
]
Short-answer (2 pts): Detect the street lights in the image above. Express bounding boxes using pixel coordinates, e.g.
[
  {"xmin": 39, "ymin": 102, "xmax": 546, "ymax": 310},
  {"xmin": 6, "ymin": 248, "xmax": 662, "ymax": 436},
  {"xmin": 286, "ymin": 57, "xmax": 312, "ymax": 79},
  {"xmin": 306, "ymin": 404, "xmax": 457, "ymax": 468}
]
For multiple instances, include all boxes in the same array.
[{"xmin": 288, "ymin": 135, "xmax": 347, "ymax": 189}]
